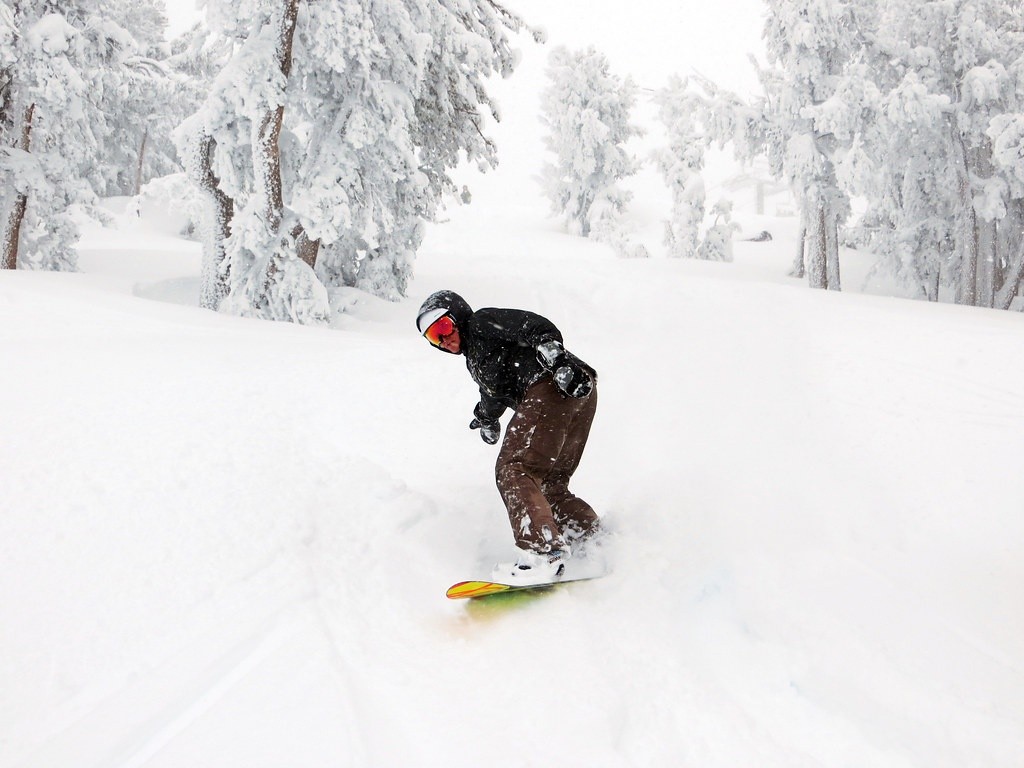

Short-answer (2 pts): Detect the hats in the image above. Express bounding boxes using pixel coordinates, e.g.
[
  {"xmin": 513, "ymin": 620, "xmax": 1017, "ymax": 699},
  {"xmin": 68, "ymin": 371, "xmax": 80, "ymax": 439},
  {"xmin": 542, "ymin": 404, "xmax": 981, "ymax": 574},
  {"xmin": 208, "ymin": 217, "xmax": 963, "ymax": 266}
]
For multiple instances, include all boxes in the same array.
[{"xmin": 419, "ymin": 309, "xmax": 449, "ymax": 337}]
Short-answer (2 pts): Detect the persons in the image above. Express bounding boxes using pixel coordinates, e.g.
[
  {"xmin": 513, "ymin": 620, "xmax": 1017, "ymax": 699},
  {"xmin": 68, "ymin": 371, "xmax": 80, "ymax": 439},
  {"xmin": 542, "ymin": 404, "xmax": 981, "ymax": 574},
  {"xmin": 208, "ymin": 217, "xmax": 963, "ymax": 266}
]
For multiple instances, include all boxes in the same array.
[{"xmin": 416, "ymin": 291, "xmax": 598, "ymax": 581}]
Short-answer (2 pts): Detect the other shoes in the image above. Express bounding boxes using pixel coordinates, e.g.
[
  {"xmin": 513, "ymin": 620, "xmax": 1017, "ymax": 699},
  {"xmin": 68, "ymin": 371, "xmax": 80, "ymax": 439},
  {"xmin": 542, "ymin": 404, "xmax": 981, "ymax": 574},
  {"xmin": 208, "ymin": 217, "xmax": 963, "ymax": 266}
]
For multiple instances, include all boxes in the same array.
[{"xmin": 498, "ymin": 552, "xmax": 565, "ymax": 587}]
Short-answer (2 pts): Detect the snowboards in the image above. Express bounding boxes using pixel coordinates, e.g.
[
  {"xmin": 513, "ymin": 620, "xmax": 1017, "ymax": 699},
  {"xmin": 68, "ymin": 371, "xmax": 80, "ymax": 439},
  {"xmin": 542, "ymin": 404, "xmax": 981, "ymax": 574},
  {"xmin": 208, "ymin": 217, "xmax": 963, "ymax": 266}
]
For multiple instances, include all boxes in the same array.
[{"xmin": 446, "ymin": 572, "xmax": 599, "ymax": 603}]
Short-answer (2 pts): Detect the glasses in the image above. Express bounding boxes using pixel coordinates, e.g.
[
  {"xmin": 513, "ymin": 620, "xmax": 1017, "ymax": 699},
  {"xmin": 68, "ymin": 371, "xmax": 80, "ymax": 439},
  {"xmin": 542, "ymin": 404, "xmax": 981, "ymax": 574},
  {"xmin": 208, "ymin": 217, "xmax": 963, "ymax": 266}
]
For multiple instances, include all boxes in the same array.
[{"xmin": 425, "ymin": 314, "xmax": 457, "ymax": 346}]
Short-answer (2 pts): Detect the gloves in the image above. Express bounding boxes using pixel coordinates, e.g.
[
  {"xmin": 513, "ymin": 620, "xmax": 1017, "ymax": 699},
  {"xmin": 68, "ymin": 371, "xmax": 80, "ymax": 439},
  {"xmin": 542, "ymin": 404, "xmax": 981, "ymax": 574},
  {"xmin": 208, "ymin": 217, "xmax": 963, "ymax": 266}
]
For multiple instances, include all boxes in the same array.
[
  {"xmin": 553, "ymin": 360, "xmax": 592, "ymax": 400},
  {"xmin": 469, "ymin": 418, "xmax": 501, "ymax": 444}
]
